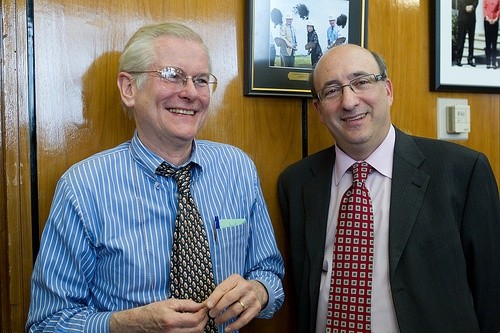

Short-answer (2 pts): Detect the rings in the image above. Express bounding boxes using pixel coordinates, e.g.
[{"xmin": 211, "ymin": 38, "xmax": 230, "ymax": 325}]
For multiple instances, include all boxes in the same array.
[{"xmin": 238, "ymin": 299, "xmax": 246, "ymax": 311}]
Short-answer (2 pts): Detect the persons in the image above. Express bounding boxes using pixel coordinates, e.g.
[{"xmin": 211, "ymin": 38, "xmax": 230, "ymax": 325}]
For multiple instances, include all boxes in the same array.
[
  {"xmin": 456, "ymin": 0, "xmax": 479, "ymax": 67},
  {"xmin": 25, "ymin": 22, "xmax": 285, "ymax": 333},
  {"xmin": 336, "ymin": 14, "xmax": 347, "ymax": 46},
  {"xmin": 482, "ymin": 0, "xmax": 500, "ymax": 69},
  {"xmin": 271, "ymin": 8, "xmax": 287, "ymax": 68},
  {"xmin": 276, "ymin": 44, "xmax": 500, "ymax": 333},
  {"xmin": 281, "ymin": 13, "xmax": 297, "ymax": 67},
  {"xmin": 327, "ymin": 16, "xmax": 339, "ymax": 51},
  {"xmin": 305, "ymin": 22, "xmax": 323, "ymax": 68}
]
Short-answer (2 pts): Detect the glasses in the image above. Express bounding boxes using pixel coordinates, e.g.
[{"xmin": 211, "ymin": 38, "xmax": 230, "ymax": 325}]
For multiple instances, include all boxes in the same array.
[
  {"xmin": 127, "ymin": 66, "xmax": 217, "ymax": 98},
  {"xmin": 316, "ymin": 74, "xmax": 384, "ymax": 102}
]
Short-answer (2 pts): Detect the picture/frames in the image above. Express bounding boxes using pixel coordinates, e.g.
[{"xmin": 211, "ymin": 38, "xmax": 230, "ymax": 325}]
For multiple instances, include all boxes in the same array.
[
  {"xmin": 244, "ymin": 0, "xmax": 367, "ymax": 100},
  {"xmin": 430, "ymin": 0, "xmax": 500, "ymax": 92}
]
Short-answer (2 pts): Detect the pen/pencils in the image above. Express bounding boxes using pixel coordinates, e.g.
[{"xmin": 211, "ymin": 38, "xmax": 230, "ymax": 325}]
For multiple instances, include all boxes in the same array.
[{"xmin": 214, "ymin": 216, "xmax": 219, "ymax": 241}]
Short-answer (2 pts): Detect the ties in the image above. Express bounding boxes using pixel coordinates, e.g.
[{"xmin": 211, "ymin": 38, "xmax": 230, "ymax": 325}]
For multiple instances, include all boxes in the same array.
[
  {"xmin": 155, "ymin": 161, "xmax": 218, "ymax": 333},
  {"xmin": 331, "ymin": 29, "xmax": 334, "ymax": 43},
  {"xmin": 290, "ymin": 26, "xmax": 293, "ymax": 40},
  {"xmin": 324, "ymin": 161, "xmax": 377, "ymax": 333}
]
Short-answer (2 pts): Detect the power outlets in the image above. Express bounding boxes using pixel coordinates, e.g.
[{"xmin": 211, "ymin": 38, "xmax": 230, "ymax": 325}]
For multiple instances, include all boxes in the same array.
[{"xmin": 438, "ymin": 97, "xmax": 471, "ymax": 141}]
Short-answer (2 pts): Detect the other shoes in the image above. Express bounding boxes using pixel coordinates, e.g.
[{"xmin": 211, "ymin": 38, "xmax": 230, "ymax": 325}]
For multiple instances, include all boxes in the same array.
[
  {"xmin": 468, "ymin": 61, "xmax": 475, "ymax": 66},
  {"xmin": 487, "ymin": 64, "xmax": 496, "ymax": 69},
  {"xmin": 457, "ymin": 60, "xmax": 462, "ymax": 66}
]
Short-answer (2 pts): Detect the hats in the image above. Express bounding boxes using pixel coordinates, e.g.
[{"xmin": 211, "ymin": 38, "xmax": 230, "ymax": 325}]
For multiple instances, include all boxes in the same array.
[
  {"xmin": 306, "ymin": 21, "xmax": 314, "ymax": 26},
  {"xmin": 286, "ymin": 15, "xmax": 294, "ymax": 18},
  {"xmin": 328, "ymin": 16, "xmax": 336, "ymax": 20}
]
